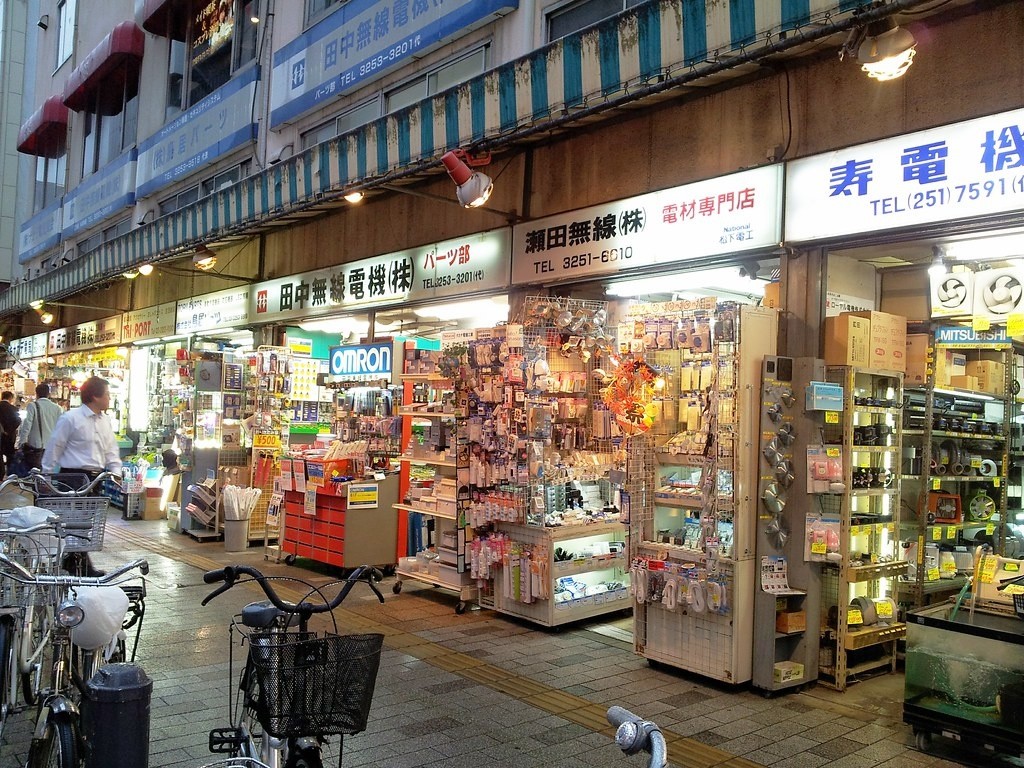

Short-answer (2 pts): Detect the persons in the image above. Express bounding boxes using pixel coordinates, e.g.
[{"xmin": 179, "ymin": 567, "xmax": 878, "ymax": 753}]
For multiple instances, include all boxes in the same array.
[
  {"xmin": 41, "ymin": 375, "xmax": 122, "ymax": 577},
  {"xmin": 14, "ymin": 383, "xmax": 64, "ymax": 477},
  {"xmin": 0, "ymin": 391, "xmax": 21, "ymax": 481}
]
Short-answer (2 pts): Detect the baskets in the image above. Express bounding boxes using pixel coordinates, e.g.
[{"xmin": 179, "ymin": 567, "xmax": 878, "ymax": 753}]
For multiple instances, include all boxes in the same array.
[
  {"xmin": 0, "ymin": 532, "xmax": 62, "ymax": 607},
  {"xmin": 36, "ymin": 495, "xmax": 111, "ymax": 553},
  {"xmin": 246, "ymin": 632, "xmax": 384, "ymax": 739}
]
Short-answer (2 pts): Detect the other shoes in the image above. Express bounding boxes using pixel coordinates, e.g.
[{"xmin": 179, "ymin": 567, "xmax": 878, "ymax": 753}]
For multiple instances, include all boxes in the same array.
[{"xmin": 60, "ymin": 557, "xmax": 106, "ymax": 577}]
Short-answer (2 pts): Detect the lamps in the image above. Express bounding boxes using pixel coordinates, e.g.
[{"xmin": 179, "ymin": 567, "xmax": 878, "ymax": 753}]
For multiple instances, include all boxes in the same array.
[
  {"xmin": 439, "ymin": 143, "xmax": 495, "ymax": 209},
  {"xmin": 122, "ymin": 269, "xmax": 139, "ymax": 279},
  {"xmin": 854, "ymin": 4, "xmax": 917, "ymax": 84},
  {"xmin": 33, "ymin": 302, "xmax": 53, "ymax": 324},
  {"xmin": 192, "ymin": 241, "xmax": 216, "ymax": 271}
]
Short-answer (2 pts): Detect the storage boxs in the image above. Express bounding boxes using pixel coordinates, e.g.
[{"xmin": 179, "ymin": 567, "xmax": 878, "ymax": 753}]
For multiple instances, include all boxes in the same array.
[
  {"xmin": 945, "ymin": 347, "xmax": 1010, "ymax": 396},
  {"xmin": 772, "ymin": 660, "xmax": 805, "ymax": 684},
  {"xmin": 436, "ymin": 476, "xmax": 476, "ymax": 589},
  {"xmin": 824, "ymin": 309, "xmax": 908, "ymax": 372},
  {"xmin": 776, "ymin": 607, "xmax": 807, "ymax": 634}
]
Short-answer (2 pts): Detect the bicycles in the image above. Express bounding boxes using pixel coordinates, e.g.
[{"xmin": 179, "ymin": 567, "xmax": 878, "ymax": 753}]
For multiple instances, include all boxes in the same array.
[
  {"xmin": 0, "ymin": 468, "xmax": 149, "ymax": 768},
  {"xmin": 195, "ymin": 564, "xmax": 386, "ymax": 768}
]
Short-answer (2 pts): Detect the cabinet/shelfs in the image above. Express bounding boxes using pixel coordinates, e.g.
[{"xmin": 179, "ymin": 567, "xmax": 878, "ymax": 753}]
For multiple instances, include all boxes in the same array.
[
  {"xmin": 898, "ymin": 320, "xmax": 1024, "ymax": 611},
  {"xmin": 813, "ymin": 365, "xmax": 902, "ymax": 692},
  {"xmin": 393, "ymin": 337, "xmax": 479, "ymax": 612}
]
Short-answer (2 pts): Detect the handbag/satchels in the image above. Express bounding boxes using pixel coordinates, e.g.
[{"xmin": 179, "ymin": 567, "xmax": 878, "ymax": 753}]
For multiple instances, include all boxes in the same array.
[{"xmin": 23, "ymin": 443, "xmax": 45, "ymax": 470}]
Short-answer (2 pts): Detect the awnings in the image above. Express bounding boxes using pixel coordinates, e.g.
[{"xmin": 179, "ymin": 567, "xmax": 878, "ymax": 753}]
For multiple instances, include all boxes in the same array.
[
  {"xmin": 16, "ymin": 96, "xmax": 67, "ymax": 152},
  {"xmin": 63, "ymin": 20, "xmax": 145, "ymax": 113},
  {"xmin": 134, "ymin": 0, "xmax": 165, "ymax": 35}
]
